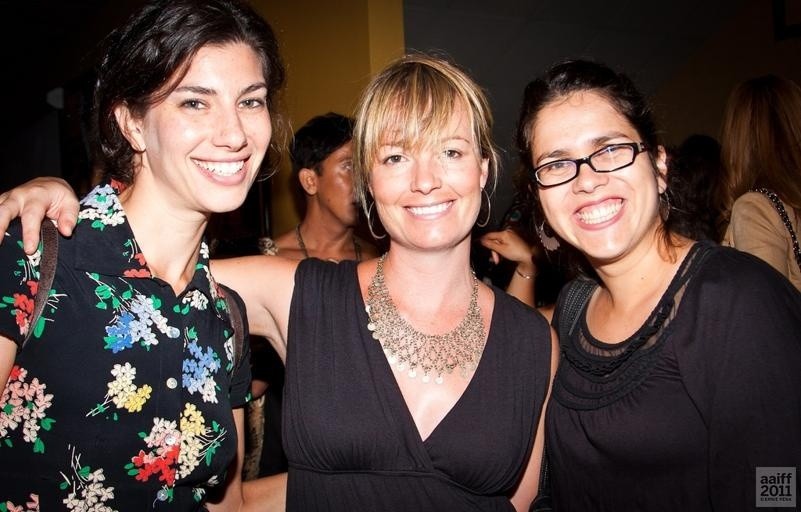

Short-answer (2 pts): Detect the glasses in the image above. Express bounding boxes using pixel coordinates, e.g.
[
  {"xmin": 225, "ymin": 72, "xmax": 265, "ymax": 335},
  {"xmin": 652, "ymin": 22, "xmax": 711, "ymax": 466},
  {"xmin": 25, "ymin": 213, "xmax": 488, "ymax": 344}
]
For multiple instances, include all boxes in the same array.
[{"xmin": 529, "ymin": 142, "xmax": 648, "ymax": 188}]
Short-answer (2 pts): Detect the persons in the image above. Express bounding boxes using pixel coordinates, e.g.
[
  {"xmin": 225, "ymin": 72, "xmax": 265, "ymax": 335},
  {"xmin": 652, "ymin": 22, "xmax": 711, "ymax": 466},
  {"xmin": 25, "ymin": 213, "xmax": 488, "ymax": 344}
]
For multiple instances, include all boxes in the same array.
[{"xmin": 0, "ymin": 0, "xmax": 801, "ymax": 512}]
